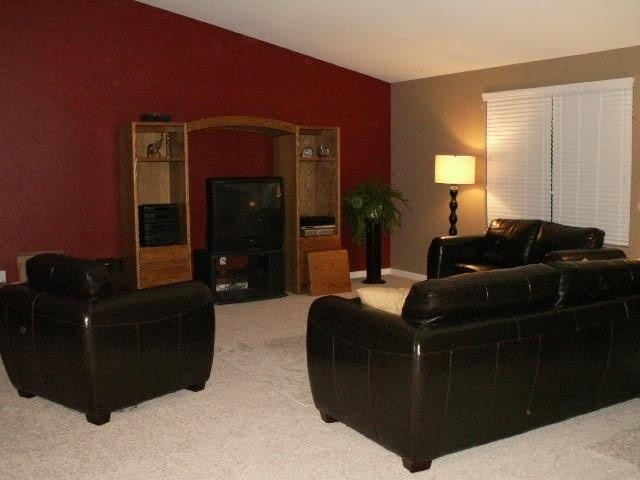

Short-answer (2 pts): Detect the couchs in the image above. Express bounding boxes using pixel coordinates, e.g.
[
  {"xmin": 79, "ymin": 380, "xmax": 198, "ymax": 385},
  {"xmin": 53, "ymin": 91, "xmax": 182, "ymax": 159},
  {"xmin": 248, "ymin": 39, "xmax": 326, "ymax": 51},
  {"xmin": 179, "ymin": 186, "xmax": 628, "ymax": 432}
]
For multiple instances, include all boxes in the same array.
[
  {"xmin": 426, "ymin": 234, "xmax": 626, "ymax": 280},
  {"xmin": 302, "ymin": 294, "xmax": 640, "ymax": 473}
]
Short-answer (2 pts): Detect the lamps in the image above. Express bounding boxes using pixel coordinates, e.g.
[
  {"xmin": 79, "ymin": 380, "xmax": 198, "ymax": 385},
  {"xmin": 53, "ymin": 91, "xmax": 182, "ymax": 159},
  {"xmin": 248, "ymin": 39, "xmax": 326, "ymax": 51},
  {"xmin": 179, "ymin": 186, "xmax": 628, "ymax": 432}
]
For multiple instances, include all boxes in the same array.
[{"xmin": 434, "ymin": 152, "xmax": 476, "ymax": 236}]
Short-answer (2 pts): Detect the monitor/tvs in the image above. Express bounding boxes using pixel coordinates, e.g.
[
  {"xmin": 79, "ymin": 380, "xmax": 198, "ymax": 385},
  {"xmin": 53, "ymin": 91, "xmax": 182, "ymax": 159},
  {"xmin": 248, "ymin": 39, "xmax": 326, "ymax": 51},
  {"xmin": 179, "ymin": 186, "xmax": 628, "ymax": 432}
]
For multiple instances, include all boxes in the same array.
[{"xmin": 205, "ymin": 176, "xmax": 285, "ymax": 254}]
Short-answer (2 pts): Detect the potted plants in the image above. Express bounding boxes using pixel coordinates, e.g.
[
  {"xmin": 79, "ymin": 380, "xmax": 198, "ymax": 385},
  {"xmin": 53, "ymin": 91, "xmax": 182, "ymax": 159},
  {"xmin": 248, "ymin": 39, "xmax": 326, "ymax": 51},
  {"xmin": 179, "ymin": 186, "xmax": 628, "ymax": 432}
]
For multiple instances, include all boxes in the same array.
[{"xmin": 342, "ymin": 175, "xmax": 413, "ymax": 284}]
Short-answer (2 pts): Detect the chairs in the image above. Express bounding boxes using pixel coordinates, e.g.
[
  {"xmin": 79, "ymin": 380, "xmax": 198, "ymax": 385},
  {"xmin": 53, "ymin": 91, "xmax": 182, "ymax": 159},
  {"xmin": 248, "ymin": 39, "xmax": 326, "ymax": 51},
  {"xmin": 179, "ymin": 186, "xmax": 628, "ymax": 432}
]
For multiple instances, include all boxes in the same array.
[{"xmin": 1, "ymin": 272, "xmax": 216, "ymax": 426}]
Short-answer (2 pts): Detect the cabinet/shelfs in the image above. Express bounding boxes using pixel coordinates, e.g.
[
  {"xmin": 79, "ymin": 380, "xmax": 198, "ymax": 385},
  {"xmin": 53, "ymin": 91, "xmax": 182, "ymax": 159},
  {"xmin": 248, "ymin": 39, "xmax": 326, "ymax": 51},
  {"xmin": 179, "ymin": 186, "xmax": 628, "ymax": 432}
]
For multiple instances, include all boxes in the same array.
[
  {"xmin": 193, "ymin": 250, "xmax": 288, "ymax": 305},
  {"xmin": 117, "ymin": 116, "xmax": 341, "ymax": 294}
]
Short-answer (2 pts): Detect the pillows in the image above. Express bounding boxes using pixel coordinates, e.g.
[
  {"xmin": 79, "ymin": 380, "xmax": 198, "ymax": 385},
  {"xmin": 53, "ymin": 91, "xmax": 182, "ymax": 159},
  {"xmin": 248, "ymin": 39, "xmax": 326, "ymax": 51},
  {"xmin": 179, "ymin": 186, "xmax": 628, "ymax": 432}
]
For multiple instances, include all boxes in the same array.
[
  {"xmin": 358, "ymin": 287, "xmax": 410, "ymax": 316},
  {"xmin": 401, "ymin": 262, "xmax": 561, "ymax": 330},
  {"xmin": 545, "ymin": 259, "xmax": 640, "ymax": 309},
  {"xmin": 527, "ymin": 221, "xmax": 605, "ymax": 264},
  {"xmin": 25, "ymin": 253, "xmax": 112, "ymax": 299},
  {"xmin": 483, "ymin": 219, "xmax": 542, "ymax": 268}
]
